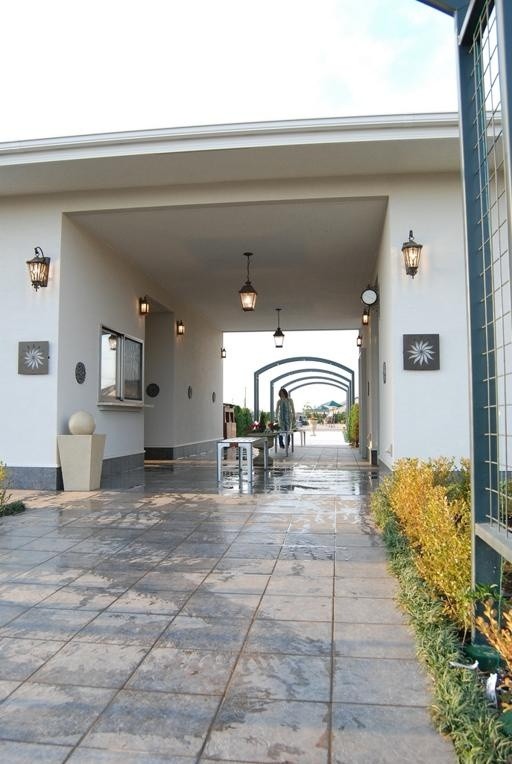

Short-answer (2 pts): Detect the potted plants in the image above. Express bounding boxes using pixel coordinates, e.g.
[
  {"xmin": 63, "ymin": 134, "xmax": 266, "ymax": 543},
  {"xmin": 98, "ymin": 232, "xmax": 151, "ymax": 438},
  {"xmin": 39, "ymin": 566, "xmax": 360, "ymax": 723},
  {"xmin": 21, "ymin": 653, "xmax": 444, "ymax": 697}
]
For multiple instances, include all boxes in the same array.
[{"xmin": 246, "ymin": 409, "xmax": 280, "ymax": 466}]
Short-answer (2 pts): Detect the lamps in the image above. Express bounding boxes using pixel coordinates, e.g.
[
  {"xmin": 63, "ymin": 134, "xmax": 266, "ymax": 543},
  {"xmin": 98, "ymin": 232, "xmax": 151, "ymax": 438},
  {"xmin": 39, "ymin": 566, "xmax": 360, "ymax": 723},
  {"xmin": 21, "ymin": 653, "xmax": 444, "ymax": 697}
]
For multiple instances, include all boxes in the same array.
[
  {"xmin": 26, "ymin": 247, "xmax": 50, "ymax": 292},
  {"xmin": 401, "ymin": 230, "xmax": 423, "ymax": 279},
  {"xmin": 273, "ymin": 308, "xmax": 284, "ymax": 348},
  {"xmin": 357, "ymin": 335, "xmax": 362, "ymax": 346},
  {"xmin": 139, "ymin": 296, "xmax": 150, "ymax": 315},
  {"xmin": 362, "ymin": 309, "xmax": 368, "ymax": 325},
  {"xmin": 239, "ymin": 252, "xmax": 258, "ymax": 311},
  {"xmin": 221, "ymin": 348, "xmax": 226, "ymax": 358},
  {"xmin": 109, "ymin": 334, "xmax": 116, "ymax": 350},
  {"xmin": 177, "ymin": 320, "xmax": 185, "ymax": 335}
]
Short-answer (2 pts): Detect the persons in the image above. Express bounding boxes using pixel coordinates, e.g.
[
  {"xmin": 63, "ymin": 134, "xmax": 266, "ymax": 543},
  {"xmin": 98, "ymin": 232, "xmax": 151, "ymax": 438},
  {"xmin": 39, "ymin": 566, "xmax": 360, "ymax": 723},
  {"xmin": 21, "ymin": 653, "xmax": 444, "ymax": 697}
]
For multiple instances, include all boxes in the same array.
[{"xmin": 274, "ymin": 387, "xmax": 295, "ymax": 449}]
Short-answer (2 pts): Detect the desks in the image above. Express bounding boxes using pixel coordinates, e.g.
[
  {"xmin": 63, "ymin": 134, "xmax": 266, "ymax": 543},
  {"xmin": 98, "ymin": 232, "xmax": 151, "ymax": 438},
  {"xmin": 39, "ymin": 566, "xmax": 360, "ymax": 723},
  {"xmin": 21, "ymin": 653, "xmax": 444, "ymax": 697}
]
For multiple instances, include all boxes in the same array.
[
  {"xmin": 217, "ymin": 437, "xmax": 268, "ymax": 482},
  {"xmin": 275, "ymin": 430, "xmax": 306, "ymax": 457}
]
